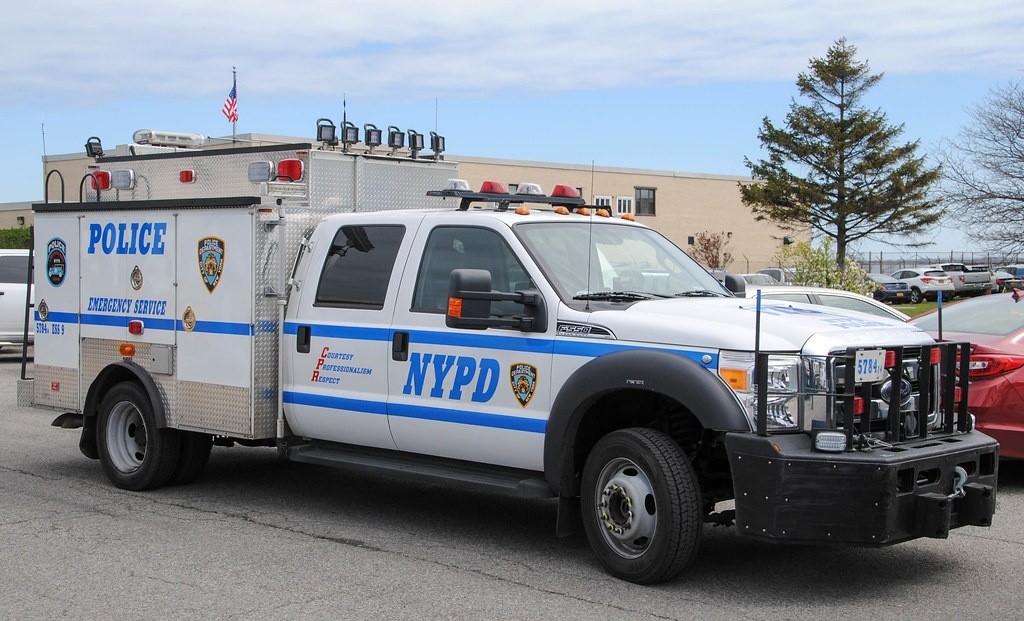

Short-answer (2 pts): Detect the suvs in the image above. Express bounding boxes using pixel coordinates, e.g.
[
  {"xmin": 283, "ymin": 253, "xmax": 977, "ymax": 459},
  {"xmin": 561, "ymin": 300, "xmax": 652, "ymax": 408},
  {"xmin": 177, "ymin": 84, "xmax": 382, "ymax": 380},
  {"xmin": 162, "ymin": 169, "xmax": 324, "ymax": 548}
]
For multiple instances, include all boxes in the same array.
[{"xmin": 890, "ymin": 267, "xmax": 955, "ymax": 304}]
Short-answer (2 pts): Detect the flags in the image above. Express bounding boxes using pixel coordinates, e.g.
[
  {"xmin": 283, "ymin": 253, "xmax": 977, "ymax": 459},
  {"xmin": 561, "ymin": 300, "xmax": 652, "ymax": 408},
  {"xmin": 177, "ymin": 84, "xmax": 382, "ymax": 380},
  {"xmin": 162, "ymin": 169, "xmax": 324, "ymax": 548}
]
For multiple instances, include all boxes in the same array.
[{"xmin": 222, "ymin": 80, "xmax": 239, "ymax": 123}]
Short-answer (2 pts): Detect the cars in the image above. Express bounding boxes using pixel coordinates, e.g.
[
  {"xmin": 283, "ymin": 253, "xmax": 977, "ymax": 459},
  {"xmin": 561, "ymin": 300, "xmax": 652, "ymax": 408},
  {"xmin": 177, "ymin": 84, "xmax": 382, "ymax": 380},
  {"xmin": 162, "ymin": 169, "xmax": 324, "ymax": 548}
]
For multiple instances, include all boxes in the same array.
[
  {"xmin": 640, "ymin": 267, "xmax": 911, "ymax": 323},
  {"xmin": 0, "ymin": 247, "xmax": 36, "ymax": 346},
  {"xmin": 991, "ymin": 262, "xmax": 1024, "ymax": 294},
  {"xmin": 864, "ymin": 273, "xmax": 912, "ymax": 305},
  {"xmin": 901, "ymin": 287, "xmax": 1024, "ymax": 486}
]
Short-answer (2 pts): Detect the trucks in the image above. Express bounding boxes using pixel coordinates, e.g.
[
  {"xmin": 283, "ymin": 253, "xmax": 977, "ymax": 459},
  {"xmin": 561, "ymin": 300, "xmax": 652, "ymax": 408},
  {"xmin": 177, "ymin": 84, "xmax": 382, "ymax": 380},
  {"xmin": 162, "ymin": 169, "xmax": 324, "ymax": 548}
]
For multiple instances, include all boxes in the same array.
[{"xmin": 18, "ymin": 118, "xmax": 1000, "ymax": 588}]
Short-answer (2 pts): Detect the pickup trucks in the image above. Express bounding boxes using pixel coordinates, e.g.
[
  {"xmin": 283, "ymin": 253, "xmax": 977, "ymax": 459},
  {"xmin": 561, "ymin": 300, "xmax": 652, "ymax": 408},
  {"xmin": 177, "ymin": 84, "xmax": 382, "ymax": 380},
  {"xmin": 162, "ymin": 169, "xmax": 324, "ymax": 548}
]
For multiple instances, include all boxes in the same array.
[{"xmin": 923, "ymin": 262, "xmax": 995, "ymax": 302}]
